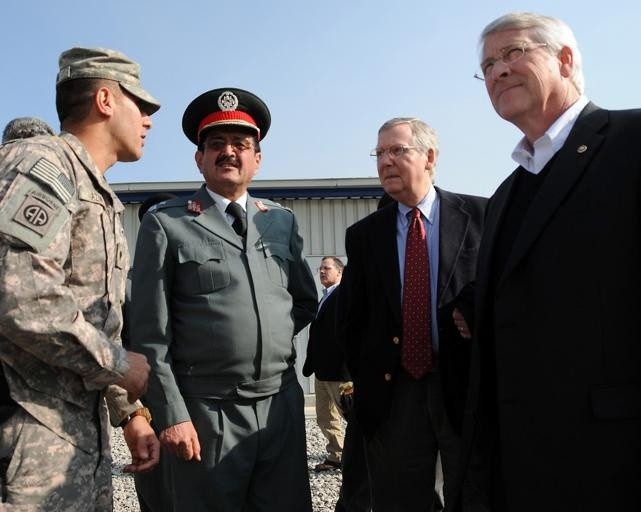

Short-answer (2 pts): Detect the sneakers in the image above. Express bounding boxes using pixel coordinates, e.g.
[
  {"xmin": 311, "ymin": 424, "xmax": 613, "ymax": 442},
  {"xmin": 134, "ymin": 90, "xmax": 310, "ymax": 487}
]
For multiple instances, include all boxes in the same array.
[{"xmin": 315, "ymin": 463, "xmax": 337, "ymax": 471}]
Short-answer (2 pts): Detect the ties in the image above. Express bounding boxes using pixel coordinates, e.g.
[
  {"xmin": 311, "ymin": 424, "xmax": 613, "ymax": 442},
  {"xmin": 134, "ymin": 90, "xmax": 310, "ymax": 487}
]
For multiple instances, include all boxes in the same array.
[
  {"xmin": 401, "ymin": 207, "xmax": 433, "ymax": 379},
  {"xmin": 225, "ymin": 202, "xmax": 247, "ymax": 237}
]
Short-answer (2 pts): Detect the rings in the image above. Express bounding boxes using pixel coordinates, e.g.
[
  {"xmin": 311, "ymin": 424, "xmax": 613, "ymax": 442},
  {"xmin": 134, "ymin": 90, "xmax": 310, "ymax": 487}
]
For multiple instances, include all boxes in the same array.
[{"xmin": 461, "ymin": 326, "xmax": 464, "ymax": 332}]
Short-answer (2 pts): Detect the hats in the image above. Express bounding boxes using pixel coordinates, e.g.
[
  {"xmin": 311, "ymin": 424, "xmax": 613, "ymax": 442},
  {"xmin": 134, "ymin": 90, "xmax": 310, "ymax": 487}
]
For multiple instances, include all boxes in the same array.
[
  {"xmin": 182, "ymin": 88, "xmax": 271, "ymax": 149},
  {"xmin": 56, "ymin": 46, "xmax": 160, "ymax": 116}
]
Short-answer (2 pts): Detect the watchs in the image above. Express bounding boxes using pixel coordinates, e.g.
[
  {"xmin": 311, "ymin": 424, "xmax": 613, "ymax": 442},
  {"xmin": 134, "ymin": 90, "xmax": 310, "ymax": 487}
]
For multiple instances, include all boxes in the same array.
[{"xmin": 121, "ymin": 407, "xmax": 154, "ymax": 430}]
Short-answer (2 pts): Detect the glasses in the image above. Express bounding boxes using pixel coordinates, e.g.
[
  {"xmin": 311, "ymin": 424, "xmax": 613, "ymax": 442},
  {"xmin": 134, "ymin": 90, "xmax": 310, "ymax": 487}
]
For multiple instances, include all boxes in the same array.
[
  {"xmin": 317, "ymin": 266, "xmax": 338, "ymax": 272},
  {"xmin": 369, "ymin": 144, "xmax": 422, "ymax": 162},
  {"xmin": 473, "ymin": 42, "xmax": 548, "ymax": 80},
  {"xmin": 200, "ymin": 136, "xmax": 257, "ymax": 153}
]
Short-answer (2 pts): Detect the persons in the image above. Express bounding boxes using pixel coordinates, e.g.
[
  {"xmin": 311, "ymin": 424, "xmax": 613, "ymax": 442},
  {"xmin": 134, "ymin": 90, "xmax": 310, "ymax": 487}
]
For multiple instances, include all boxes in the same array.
[
  {"xmin": 0, "ymin": 46, "xmax": 162, "ymax": 512},
  {"xmin": 462, "ymin": 10, "xmax": 640, "ymax": 511},
  {"xmin": 1, "ymin": 116, "xmax": 57, "ymax": 144},
  {"xmin": 333, "ymin": 117, "xmax": 493, "ymax": 512},
  {"xmin": 118, "ymin": 191, "xmax": 181, "ymax": 352},
  {"xmin": 305, "ymin": 255, "xmax": 354, "ymax": 475},
  {"xmin": 127, "ymin": 88, "xmax": 321, "ymax": 511}
]
